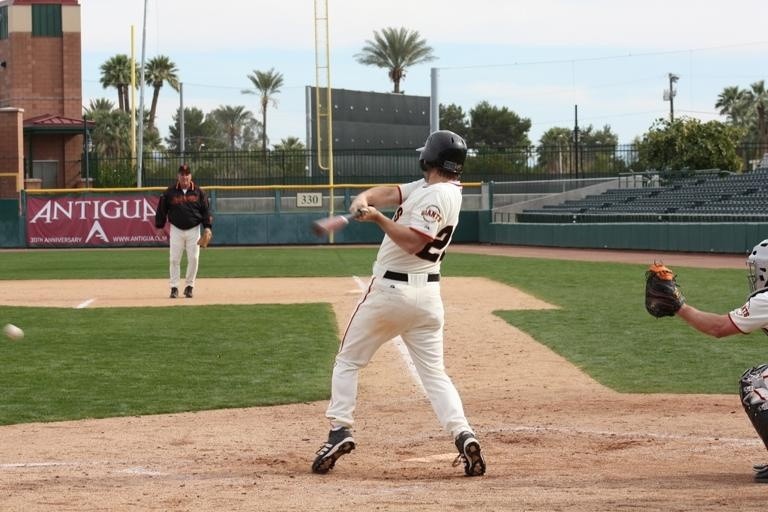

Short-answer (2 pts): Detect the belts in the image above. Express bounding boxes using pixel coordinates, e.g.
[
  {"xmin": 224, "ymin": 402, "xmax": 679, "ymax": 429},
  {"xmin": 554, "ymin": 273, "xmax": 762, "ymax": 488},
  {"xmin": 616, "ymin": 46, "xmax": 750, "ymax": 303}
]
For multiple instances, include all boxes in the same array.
[{"xmin": 381, "ymin": 269, "xmax": 441, "ymax": 283}]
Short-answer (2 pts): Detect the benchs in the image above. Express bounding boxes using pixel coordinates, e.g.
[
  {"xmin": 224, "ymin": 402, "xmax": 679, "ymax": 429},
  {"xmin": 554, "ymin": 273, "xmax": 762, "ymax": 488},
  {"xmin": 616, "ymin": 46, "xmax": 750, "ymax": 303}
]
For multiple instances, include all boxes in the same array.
[{"xmin": 506, "ymin": 166, "xmax": 767, "ymax": 228}]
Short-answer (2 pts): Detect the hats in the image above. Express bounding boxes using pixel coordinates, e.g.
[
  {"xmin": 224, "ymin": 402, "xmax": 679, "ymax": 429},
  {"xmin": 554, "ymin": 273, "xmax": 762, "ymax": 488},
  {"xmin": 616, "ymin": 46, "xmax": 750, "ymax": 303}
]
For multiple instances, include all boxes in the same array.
[{"xmin": 178, "ymin": 164, "xmax": 192, "ymax": 174}]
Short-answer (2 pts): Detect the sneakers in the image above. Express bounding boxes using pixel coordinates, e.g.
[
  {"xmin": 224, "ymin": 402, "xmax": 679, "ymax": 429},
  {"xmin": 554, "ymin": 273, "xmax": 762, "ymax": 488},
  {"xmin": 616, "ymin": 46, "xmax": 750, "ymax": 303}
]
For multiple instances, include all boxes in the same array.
[
  {"xmin": 311, "ymin": 425, "xmax": 358, "ymax": 476},
  {"xmin": 170, "ymin": 287, "xmax": 179, "ymax": 298},
  {"xmin": 752, "ymin": 460, "xmax": 768, "ymax": 484},
  {"xmin": 451, "ymin": 429, "xmax": 487, "ymax": 478},
  {"xmin": 184, "ymin": 285, "xmax": 194, "ymax": 298}
]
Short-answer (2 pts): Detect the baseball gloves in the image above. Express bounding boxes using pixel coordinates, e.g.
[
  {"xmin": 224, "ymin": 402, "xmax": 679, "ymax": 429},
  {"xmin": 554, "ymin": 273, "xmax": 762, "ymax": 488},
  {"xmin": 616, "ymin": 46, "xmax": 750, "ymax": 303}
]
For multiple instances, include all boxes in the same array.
[
  {"xmin": 197, "ymin": 228, "xmax": 212, "ymax": 248},
  {"xmin": 644, "ymin": 261, "xmax": 686, "ymax": 318}
]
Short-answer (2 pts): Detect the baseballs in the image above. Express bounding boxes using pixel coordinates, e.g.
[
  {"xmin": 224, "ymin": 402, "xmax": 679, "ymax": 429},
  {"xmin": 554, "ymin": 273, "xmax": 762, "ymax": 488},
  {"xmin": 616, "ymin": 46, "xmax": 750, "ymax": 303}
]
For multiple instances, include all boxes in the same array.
[
  {"xmin": 5, "ymin": 324, "xmax": 24, "ymax": 340},
  {"xmin": 312, "ymin": 205, "xmax": 374, "ymax": 237}
]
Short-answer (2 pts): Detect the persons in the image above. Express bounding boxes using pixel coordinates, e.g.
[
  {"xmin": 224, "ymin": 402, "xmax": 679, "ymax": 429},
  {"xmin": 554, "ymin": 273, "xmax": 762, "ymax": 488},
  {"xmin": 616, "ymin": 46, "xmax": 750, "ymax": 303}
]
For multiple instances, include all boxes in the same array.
[
  {"xmin": 155, "ymin": 163, "xmax": 213, "ymax": 299},
  {"xmin": 645, "ymin": 238, "xmax": 768, "ymax": 482},
  {"xmin": 311, "ymin": 128, "xmax": 487, "ymax": 478}
]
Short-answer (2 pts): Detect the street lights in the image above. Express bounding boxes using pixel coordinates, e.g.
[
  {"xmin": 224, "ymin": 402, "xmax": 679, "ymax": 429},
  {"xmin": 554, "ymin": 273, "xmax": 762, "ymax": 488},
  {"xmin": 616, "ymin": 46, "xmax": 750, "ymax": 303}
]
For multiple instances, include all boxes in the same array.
[
  {"xmin": 558, "ymin": 134, "xmax": 566, "ymax": 177},
  {"xmin": 83, "ymin": 129, "xmax": 95, "ymax": 188},
  {"xmin": 665, "ymin": 70, "xmax": 681, "ymax": 123},
  {"xmin": 571, "ymin": 125, "xmax": 582, "ymax": 188}
]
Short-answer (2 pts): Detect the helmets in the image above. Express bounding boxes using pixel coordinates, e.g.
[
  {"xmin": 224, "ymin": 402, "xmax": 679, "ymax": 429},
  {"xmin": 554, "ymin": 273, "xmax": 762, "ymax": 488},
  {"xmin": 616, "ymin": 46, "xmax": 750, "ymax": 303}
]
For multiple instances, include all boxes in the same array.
[
  {"xmin": 416, "ymin": 129, "xmax": 469, "ymax": 174},
  {"xmin": 746, "ymin": 238, "xmax": 768, "ymax": 290}
]
